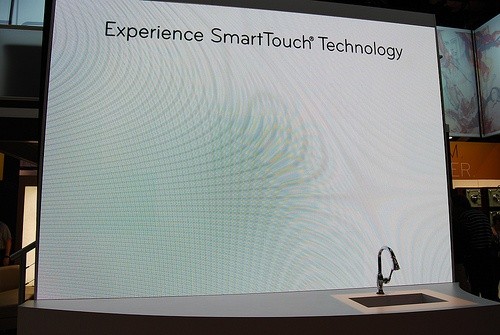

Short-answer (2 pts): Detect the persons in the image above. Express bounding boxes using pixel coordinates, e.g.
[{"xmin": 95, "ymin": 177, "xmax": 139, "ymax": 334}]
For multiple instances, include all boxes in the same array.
[
  {"xmin": 491, "ymin": 214, "xmax": 500, "ymax": 249},
  {"xmin": 452, "ymin": 193, "xmax": 500, "ymax": 302},
  {"xmin": 0, "ymin": 220, "xmax": 15, "ymax": 266}
]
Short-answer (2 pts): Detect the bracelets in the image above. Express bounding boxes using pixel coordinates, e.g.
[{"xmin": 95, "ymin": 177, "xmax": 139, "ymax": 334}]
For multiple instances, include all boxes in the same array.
[{"xmin": 4, "ymin": 256, "xmax": 10, "ymax": 258}]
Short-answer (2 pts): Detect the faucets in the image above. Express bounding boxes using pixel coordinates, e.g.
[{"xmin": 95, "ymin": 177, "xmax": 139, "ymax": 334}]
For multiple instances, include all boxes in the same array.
[{"xmin": 376, "ymin": 246, "xmax": 400, "ymax": 294}]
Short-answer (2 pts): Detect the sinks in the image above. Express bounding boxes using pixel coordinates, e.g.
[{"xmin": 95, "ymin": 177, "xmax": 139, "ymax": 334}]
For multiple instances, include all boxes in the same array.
[{"xmin": 348, "ymin": 292, "xmax": 448, "ymax": 308}]
